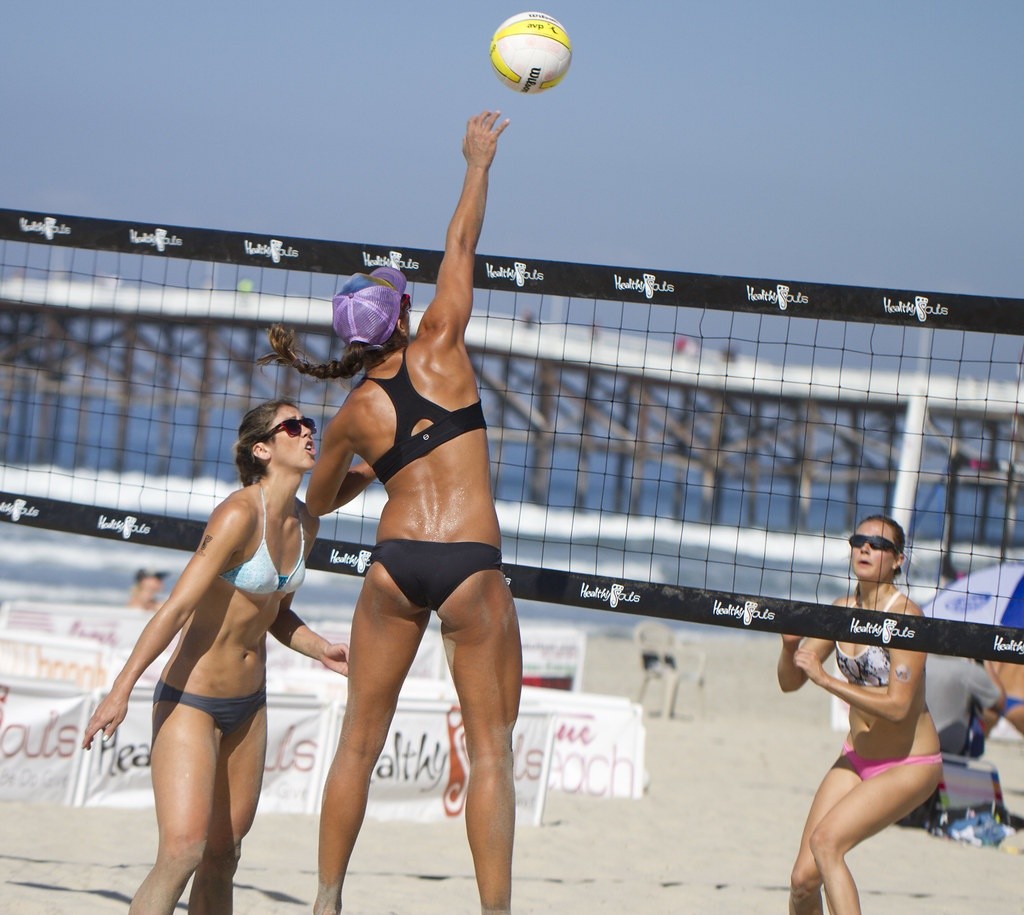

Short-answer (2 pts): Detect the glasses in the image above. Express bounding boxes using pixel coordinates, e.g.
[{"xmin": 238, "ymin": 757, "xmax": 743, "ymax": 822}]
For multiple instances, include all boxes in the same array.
[
  {"xmin": 848, "ymin": 534, "xmax": 899, "ymax": 556},
  {"xmin": 254, "ymin": 417, "xmax": 317, "ymax": 445}
]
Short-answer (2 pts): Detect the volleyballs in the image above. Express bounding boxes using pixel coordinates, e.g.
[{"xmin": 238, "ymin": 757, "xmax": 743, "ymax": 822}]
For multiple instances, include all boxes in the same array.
[{"xmin": 488, "ymin": 11, "xmax": 572, "ymax": 94}]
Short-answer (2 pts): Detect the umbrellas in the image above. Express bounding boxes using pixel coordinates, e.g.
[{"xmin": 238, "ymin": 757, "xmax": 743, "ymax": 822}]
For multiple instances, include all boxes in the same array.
[{"xmin": 921, "ymin": 561, "xmax": 1024, "ymax": 629}]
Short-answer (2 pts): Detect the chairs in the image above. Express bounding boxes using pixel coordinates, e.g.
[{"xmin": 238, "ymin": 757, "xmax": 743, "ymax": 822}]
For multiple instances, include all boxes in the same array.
[{"xmin": 634, "ymin": 623, "xmax": 706, "ymax": 720}]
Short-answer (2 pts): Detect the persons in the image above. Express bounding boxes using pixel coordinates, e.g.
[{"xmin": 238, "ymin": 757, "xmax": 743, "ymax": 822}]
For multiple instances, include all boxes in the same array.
[
  {"xmin": 124, "ymin": 570, "xmax": 167, "ymax": 612},
  {"xmin": 777, "ymin": 514, "xmax": 944, "ymax": 915},
  {"xmin": 268, "ymin": 109, "xmax": 522, "ymax": 915},
  {"xmin": 81, "ymin": 399, "xmax": 351, "ymax": 915},
  {"xmin": 924, "ymin": 652, "xmax": 1024, "ymax": 760}
]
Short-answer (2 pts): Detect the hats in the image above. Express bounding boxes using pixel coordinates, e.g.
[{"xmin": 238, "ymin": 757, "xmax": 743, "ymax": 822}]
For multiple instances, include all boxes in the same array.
[{"xmin": 330, "ymin": 266, "xmax": 410, "ymax": 347}]
